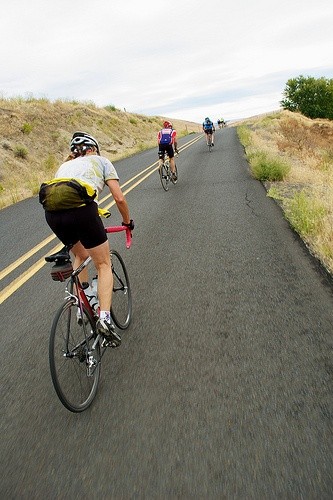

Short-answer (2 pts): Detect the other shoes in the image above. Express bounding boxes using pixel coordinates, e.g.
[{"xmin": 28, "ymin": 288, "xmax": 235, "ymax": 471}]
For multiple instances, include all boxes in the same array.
[
  {"xmin": 95, "ymin": 318, "xmax": 122, "ymax": 348},
  {"xmin": 76, "ymin": 309, "xmax": 98, "ymax": 325},
  {"xmin": 211, "ymin": 142, "xmax": 214, "ymax": 146},
  {"xmin": 170, "ymin": 173, "xmax": 177, "ymax": 180}
]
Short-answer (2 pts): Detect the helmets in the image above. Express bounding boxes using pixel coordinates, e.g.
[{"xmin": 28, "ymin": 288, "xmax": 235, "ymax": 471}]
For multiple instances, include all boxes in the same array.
[
  {"xmin": 70, "ymin": 131, "xmax": 101, "ymax": 156},
  {"xmin": 205, "ymin": 117, "xmax": 209, "ymax": 120},
  {"xmin": 163, "ymin": 121, "xmax": 172, "ymax": 128}
]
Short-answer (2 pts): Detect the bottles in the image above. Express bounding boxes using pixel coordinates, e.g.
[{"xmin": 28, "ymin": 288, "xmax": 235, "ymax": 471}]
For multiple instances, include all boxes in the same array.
[
  {"xmin": 91, "ymin": 275, "xmax": 98, "ymax": 297},
  {"xmin": 81, "ymin": 282, "xmax": 98, "ymax": 310}
]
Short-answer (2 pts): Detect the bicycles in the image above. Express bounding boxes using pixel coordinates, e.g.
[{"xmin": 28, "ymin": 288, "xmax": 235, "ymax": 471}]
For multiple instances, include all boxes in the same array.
[
  {"xmin": 42, "ymin": 223, "xmax": 134, "ymax": 410},
  {"xmin": 158, "ymin": 147, "xmax": 180, "ymax": 191},
  {"xmin": 207, "ymin": 132, "xmax": 213, "ymax": 152}
]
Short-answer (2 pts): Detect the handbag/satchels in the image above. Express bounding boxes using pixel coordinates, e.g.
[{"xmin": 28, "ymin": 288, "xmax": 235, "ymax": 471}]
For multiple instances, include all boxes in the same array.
[{"xmin": 39, "ymin": 179, "xmax": 111, "ymax": 218}]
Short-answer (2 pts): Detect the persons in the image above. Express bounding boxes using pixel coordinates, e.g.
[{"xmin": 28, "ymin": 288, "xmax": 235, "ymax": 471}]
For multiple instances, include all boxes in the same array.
[
  {"xmin": 158, "ymin": 121, "xmax": 178, "ymax": 181},
  {"xmin": 217, "ymin": 118, "xmax": 225, "ymax": 128},
  {"xmin": 39, "ymin": 131, "xmax": 134, "ymax": 347},
  {"xmin": 203, "ymin": 117, "xmax": 215, "ymax": 146}
]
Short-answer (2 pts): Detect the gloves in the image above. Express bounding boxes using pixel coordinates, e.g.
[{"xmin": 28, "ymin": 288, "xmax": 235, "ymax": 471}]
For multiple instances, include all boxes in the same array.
[{"xmin": 121, "ymin": 218, "xmax": 135, "ymax": 230}]
